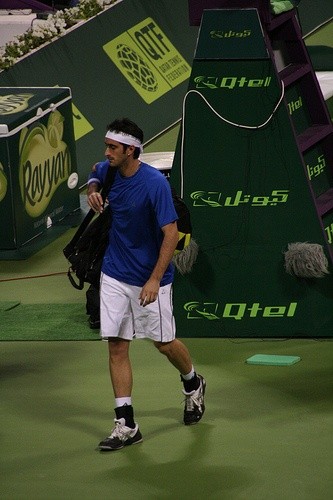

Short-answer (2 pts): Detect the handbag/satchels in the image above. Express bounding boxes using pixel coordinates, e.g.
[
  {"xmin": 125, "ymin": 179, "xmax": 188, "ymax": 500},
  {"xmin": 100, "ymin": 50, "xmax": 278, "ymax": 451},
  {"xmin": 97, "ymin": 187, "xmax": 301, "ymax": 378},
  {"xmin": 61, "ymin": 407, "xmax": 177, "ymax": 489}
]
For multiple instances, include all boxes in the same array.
[{"xmin": 63, "ymin": 195, "xmax": 192, "ymax": 291}]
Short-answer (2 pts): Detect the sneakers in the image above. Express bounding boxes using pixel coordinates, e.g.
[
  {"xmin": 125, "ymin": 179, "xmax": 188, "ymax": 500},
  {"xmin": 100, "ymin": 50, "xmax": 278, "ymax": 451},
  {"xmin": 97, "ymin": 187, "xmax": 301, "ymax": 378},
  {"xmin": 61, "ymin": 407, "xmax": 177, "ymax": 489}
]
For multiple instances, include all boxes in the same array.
[
  {"xmin": 98, "ymin": 418, "xmax": 145, "ymax": 451},
  {"xmin": 180, "ymin": 371, "xmax": 206, "ymax": 425}
]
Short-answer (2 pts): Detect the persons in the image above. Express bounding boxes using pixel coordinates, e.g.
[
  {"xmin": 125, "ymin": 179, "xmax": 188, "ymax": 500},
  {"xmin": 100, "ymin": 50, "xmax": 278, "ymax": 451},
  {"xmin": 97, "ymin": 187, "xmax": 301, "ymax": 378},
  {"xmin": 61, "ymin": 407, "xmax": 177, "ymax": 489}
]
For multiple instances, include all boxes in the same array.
[{"xmin": 86, "ymin": 118, "xmax": 206, "ymax": 448}]
